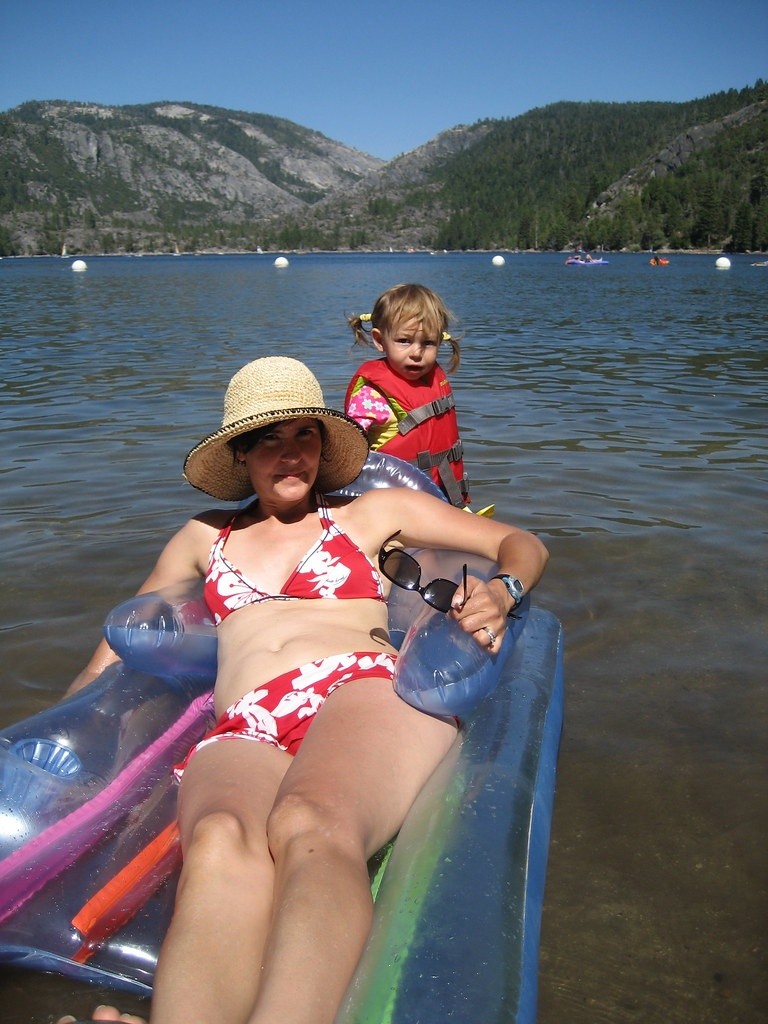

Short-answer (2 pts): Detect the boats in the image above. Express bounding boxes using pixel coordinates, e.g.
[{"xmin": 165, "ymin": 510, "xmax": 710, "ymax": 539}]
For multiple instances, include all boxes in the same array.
[
  {"xmin": 650, "ymin": 258, "xmax": 670, "ymax": 266},
  {"xmin": 0, "ymin": 450, "xmax": 567, "ymax": 1022},
  {"xmin": 565, "ymin": 258, "xmax": 609, "ymax": 266}
]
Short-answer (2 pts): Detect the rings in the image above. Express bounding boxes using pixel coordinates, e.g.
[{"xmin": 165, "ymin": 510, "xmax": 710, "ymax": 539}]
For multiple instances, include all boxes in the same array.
[{"xmin": 485, "ymin": 629, "xmax": 496, "ymax": 643}]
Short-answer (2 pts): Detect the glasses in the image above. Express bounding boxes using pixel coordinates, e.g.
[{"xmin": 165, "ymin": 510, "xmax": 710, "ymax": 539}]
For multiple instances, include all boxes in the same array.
[{"xmin": 379, "ymin": 529, "xmax": 469, "ymax": 615}]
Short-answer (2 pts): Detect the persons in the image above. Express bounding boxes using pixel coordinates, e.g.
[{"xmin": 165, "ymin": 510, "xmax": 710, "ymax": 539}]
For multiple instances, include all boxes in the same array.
[
  {"xmin": 345, "ymin": 283, "xmax": 495, "ymax": 518},
  {"xmin": 654, "ymin": 256, "xmax": 660, "ymax": 265},
  {"xmin": 568, "ymin": 253, "xmax": 598, "ymax": 262},
  {"xmin": 54, "ymin": 356, "xmax": 549, "ymax": 1024}
]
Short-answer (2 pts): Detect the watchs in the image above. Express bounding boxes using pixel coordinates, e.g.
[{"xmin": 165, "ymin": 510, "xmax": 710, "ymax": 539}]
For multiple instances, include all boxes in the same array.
[{"xmin": 489, "ymin": 574, "xmax": 524, "ymax": 610}]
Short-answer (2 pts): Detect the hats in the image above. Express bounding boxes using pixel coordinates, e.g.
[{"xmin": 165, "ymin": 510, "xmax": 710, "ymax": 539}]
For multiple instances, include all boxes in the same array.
[{"xmin": 182, "ymin": 358, "xmax": 369, "ymax": 503}]
